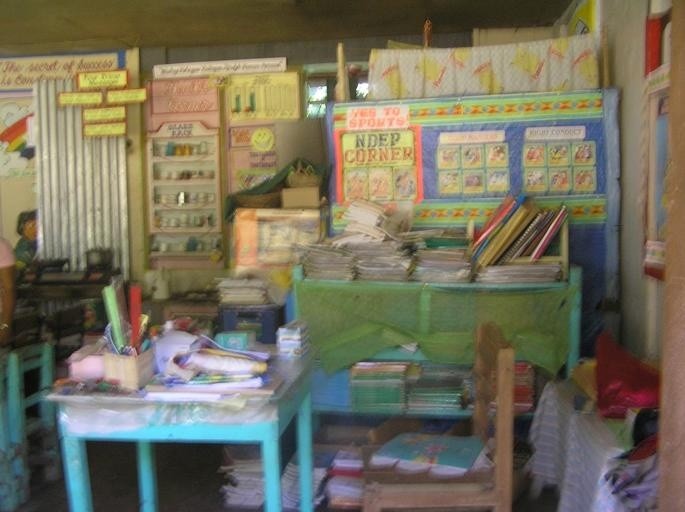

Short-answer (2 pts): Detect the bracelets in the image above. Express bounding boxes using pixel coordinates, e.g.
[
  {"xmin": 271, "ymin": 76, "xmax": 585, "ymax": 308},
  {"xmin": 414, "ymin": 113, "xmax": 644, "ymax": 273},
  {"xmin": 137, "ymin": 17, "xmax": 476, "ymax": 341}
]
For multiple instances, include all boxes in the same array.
[{"xmin": 1, "ymin": 321, "xmax": 12, "ymax": 329}]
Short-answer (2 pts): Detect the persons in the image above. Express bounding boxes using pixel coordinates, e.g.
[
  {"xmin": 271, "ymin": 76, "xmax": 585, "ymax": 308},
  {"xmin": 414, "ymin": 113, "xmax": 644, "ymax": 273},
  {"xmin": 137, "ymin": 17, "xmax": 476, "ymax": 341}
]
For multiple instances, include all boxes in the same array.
[
  {"xmin": 12, "ymin": 209, "xmax": 40, "ymax": 284},
  {"xmin": 1, "ymin": 235, "xmax": 16, "ymax": 349}
]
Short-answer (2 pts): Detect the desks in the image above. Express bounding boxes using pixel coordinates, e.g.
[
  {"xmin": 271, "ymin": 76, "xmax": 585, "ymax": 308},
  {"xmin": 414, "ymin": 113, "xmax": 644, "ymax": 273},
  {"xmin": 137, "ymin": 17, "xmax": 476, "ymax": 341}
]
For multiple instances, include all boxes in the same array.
[
  {"xmin": 43, "ymin": 341, "xmax": 321, "ymax": 512},
  {"xmin": 526, "ymin": 380, "xmax": 660, "ymax": 511}
]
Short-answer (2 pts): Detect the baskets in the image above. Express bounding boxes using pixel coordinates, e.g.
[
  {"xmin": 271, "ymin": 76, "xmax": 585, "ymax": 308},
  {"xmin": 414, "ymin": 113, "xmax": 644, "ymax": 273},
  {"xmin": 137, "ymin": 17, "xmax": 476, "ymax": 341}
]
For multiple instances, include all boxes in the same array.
[
  {"xmin": 231, "ymin": 193, "xmax": 280, "ymax": 208},
  {"xmin": 286, "ymin": 164, "xmax": 322, "ymax": 188}
]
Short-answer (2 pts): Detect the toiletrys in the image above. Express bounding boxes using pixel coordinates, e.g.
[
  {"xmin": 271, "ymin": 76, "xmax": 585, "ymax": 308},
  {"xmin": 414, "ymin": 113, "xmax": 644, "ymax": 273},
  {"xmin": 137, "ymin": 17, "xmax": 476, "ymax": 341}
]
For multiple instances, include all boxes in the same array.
[{"xmin": 150, "ymin": 138, "xmax": 221, "ymax": 195}]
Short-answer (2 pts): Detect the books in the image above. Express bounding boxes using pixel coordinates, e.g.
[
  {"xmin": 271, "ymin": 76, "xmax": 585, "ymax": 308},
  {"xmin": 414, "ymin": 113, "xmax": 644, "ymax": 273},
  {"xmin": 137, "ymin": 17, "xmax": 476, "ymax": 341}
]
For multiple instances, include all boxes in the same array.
[
  {"xmin": 220, "ymin": 276, "xmax": 270, "ymax": 306},
  {"xmin": 301, "ymin": 197, "xmax": 568, "ymax": 284},
  {"xmin": 349, "ymin": 358, "xmax": 534, "ymax": 414},
  {"xmin": 221, "ymin": 430, "xmax": 486, "ymax": 512},
  {"xmin": 144, "ymin": 334, "xmax": 286, "ymax": 405}
]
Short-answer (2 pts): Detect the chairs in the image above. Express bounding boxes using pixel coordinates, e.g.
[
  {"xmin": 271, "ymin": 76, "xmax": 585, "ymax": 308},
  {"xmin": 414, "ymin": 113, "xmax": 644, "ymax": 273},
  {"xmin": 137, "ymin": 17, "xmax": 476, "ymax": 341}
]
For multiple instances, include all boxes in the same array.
[
  {"xmin": 0, "ymin": 341, "xmax": 61, "ymax": 512},
  {"xmin": 361, "ymin": 323, "xmax": 518, "ymax": 511}
]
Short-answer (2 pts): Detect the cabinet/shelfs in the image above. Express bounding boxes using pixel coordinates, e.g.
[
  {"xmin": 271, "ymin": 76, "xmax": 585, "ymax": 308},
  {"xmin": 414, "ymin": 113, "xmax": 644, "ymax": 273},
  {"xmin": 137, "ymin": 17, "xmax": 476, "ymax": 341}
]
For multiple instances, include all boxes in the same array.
[
  {"xmin": 165, "ymin": 268, "xmax": 580, "ymax": 420},
  {"xmin": 145, "ymin": 117, "xmax": 228, "ymax": 272}
]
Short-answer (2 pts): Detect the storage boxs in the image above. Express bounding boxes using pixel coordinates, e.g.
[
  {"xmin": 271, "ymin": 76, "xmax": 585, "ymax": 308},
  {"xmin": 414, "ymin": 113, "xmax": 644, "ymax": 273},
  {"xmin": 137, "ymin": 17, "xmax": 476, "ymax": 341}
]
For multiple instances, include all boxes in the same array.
[{"xmin": 278, "ymin": 183, "xmax": 323, "ymax": 210}]
